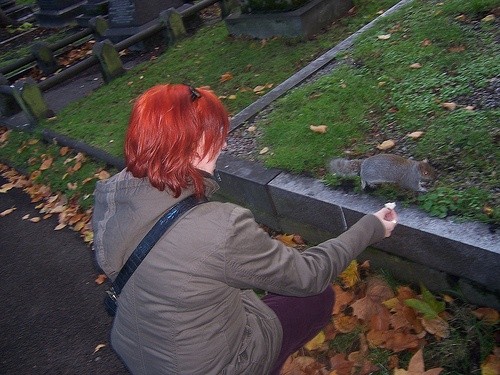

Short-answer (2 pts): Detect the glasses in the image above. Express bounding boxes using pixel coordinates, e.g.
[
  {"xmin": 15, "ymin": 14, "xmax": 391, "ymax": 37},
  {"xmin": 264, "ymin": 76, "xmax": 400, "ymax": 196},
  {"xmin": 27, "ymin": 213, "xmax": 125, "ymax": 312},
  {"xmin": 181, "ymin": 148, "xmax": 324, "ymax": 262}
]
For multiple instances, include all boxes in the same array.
[{"xmin": 180, "ymin": 83, "xmax": 201, "ymax": 103}]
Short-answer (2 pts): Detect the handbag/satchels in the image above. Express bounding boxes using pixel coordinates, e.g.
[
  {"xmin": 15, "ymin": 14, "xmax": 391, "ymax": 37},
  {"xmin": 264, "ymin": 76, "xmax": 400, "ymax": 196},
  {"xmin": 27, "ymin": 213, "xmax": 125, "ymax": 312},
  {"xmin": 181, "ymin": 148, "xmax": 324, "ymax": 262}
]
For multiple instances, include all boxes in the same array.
[{"xmin": 103, "ymin": 289, "xmax": 118, "ymax": 316}]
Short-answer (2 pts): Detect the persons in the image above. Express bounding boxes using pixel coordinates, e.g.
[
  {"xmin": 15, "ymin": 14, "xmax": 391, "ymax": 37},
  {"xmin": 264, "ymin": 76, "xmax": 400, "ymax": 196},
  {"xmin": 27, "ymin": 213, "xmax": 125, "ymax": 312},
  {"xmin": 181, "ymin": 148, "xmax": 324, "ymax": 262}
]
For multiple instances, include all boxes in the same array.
[{"xmin": 91, "ymin": 84, "xmax": 399, "ymax": 375}]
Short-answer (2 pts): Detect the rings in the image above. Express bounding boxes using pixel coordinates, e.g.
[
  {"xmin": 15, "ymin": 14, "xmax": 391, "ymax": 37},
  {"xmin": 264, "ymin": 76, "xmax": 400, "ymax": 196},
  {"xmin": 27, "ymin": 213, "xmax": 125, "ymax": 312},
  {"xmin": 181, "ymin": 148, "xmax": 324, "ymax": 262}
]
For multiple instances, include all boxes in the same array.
[{"xmin": 391, "ymin": 220, "xmax": 397, "ymax": 224}]
